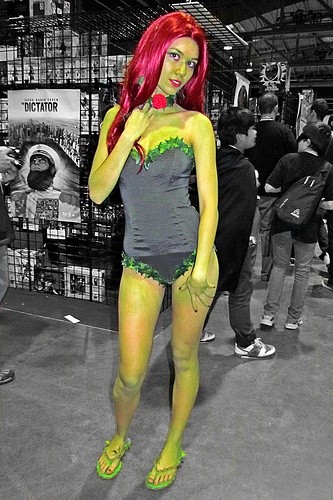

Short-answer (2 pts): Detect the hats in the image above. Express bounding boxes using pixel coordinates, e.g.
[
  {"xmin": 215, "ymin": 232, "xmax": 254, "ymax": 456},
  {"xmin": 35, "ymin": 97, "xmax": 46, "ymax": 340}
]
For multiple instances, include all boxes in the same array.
[{"xmin": 302, "ymin": 121, "xmax": 332, "ymax": 151}]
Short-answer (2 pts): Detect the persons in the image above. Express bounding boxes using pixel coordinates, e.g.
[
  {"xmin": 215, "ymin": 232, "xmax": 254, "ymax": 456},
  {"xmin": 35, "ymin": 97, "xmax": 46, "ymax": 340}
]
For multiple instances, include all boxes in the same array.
[
  {"xmin": 88, "ymin": 13, "xmax": 219, "ymax": 490},
  {"xmin": 9, "ymin": 144, "xmax": 81, "ymax": 223},
  {"xmin": 0, "ymin": 140, "xmax": 16, "ymax": 385},
  {"xmin": 199, "ymin": 107, "xmax": 276, "ymax": 357},
  {"xmin": 260, "ymin": 123, "xmax": 333, "ymax": 330},
  {"xmin": 241, "ymin": 94, "xmax": 298, "ymax": 282},
  {"xmin": 305, "ymin": 99, "xmax": 333, "ymax": 290}
]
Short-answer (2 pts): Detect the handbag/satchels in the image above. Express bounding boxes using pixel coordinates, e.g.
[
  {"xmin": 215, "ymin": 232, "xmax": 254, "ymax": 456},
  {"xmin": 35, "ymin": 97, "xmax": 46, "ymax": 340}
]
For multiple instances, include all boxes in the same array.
[{"xmin": 275, "ymin": 178, "xmax": 326, "ymax": 227}]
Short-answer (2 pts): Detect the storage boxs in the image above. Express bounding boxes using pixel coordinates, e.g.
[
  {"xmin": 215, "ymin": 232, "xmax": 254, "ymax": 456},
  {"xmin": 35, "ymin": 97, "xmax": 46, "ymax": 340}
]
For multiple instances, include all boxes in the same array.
[
  {"xmin": 63, "ymin": 265, "xmax": 106, "ymax": 303},
  {"xmin": 0, "ymin": 0, "xmax": 133, "ymax": 83}
]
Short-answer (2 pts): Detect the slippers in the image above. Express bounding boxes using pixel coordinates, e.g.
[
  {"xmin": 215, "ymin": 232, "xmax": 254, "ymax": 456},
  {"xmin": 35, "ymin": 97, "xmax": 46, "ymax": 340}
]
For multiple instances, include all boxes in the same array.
[
  {"xmin": 97, "ymin": 436, "xmax": 131, "ymax": 480},
  {"xmin": 146, "ymin": 448, "xmax": 184, "ymax": 490}
]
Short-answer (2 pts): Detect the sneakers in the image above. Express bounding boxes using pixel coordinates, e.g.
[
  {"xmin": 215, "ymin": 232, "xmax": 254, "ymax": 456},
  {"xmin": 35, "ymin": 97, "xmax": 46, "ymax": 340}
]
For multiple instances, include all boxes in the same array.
[
  {"xmin": 285, "ymin": 319, "xmax": 304, "ymax": 329},
  {"xmin": 199, "ymin": 333, "xmax": 215, "ymax": 342},
  {"xmin": 234, "ymin": 337, "xmax": 276, "ymax": 358},
  {"xmin": 0, "ymin": 369, "xmax": 13, "ymax": 383},
  {"xmin": 260, "ymin": 315, "xmax": 277, "ymax": 327}
]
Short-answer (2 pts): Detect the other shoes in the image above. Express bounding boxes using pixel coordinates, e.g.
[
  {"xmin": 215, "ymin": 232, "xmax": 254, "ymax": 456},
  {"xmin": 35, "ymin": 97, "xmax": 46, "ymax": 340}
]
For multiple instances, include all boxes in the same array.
[
  {"xmin": 261, "ymin": 275, "xmax": 270, "ymax": 281},
  {"xmin": 322, "ymin": 280, "xmax": 333, "ymax": 291},
  {"xmin": 289, "ymin": 258, "xmax": 294, "ymax": 265},
  {"xmin": 327, "ymin": 265, "xmax": 332, "ymax": 274}
]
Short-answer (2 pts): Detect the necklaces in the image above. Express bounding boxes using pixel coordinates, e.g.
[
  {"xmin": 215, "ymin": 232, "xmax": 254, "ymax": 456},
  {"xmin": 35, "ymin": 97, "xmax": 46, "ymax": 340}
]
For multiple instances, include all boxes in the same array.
[{"xmin": 146, "ymin": 94, "xmax": 176, "ymax": 112}]
